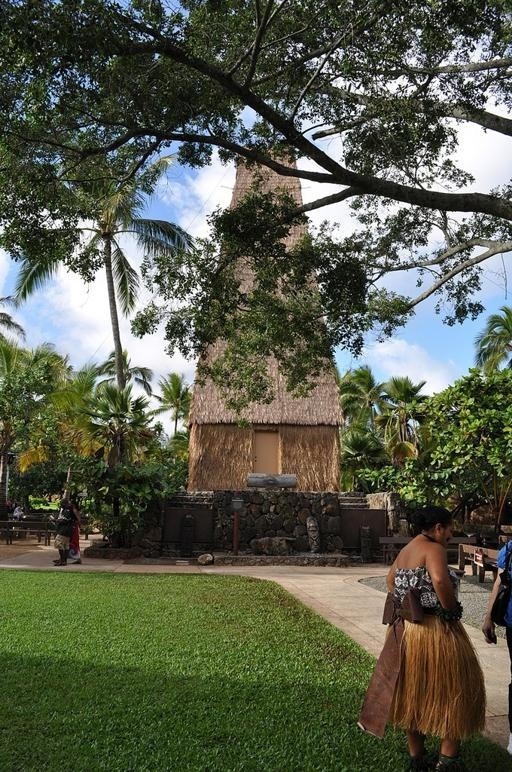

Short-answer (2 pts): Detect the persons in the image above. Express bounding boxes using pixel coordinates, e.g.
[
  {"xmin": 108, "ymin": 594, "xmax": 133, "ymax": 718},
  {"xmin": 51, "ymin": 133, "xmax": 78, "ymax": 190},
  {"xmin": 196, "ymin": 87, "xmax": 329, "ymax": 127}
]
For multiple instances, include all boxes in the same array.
[
  {"xmin": 52, "ymin": 498, "xmax": 82, "ymax": 564},
  {"xmin": 54, "ymin": 507, "xmax": 75, "ymax": 566},
  {"xmin": 355, "ymin": 506, "xmax": 486, "ymax": 771},
  {"xmin": 14, "ymin": 504, "xmax": 25, "ymax": 521},
  {"xmin": 481, "ymin": 540, "xmax": 511, "ymax": 757}
]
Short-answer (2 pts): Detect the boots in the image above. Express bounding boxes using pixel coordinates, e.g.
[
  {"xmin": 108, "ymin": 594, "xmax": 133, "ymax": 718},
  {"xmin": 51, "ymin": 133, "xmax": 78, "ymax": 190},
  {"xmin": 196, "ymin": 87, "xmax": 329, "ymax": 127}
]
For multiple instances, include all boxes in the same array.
[{"xmin": 51, "ymin": 549, "xmax": 70, "ymax": 566}]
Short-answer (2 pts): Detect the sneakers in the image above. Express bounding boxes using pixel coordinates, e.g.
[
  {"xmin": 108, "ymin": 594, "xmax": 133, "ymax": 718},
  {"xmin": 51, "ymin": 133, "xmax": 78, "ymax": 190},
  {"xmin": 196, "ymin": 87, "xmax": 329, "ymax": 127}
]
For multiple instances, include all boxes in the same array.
[{"xmin": 409, "ymin": 753, "xmax": 468, "ymax": 771}]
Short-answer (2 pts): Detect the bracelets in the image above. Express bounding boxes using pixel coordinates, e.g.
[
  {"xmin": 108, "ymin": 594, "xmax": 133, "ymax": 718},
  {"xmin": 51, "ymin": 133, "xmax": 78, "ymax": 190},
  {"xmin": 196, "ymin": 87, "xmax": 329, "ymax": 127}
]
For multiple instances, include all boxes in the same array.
[{"xmin": 436, "ymin": 600, "xmax": 465, "ymax": 624}]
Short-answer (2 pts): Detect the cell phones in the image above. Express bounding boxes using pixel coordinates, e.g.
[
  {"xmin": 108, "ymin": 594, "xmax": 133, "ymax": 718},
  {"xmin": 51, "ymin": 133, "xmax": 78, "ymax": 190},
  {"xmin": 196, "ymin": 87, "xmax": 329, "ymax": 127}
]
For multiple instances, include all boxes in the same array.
[{"xmin": 487, "ymin": 629, "xmax": 496, "ymax": 643}]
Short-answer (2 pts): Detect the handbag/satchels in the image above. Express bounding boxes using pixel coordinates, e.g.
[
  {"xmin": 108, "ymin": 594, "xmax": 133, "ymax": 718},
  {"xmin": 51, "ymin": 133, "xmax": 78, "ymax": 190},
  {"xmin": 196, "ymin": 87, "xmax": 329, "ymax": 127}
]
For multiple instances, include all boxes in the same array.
[{"xmin": 491, "ymin": 541, "xmax": 512, "ymax": 627}]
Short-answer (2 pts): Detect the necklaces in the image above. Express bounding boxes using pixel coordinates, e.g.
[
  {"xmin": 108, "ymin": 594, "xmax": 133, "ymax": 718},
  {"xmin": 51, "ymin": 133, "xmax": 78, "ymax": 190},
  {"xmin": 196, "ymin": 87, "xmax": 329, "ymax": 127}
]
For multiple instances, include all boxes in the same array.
[{"xmin": 419, "ymin": 531, "xmax": 436, "ymax": 543}]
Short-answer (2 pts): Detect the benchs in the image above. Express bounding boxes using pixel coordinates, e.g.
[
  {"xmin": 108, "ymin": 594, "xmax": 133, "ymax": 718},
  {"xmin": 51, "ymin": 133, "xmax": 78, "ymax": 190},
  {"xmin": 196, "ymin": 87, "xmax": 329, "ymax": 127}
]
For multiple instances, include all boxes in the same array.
[
  {"xmin": 458, "ymin": 544, "xmax": 500, "ymax": 583},
  {"xmin": 378, "ymin": 536, "xmax": 477, "ymax": 566},
  {"xmin": 0, "ymin": 521, "xmax": 88, "ymax": 546}
]
